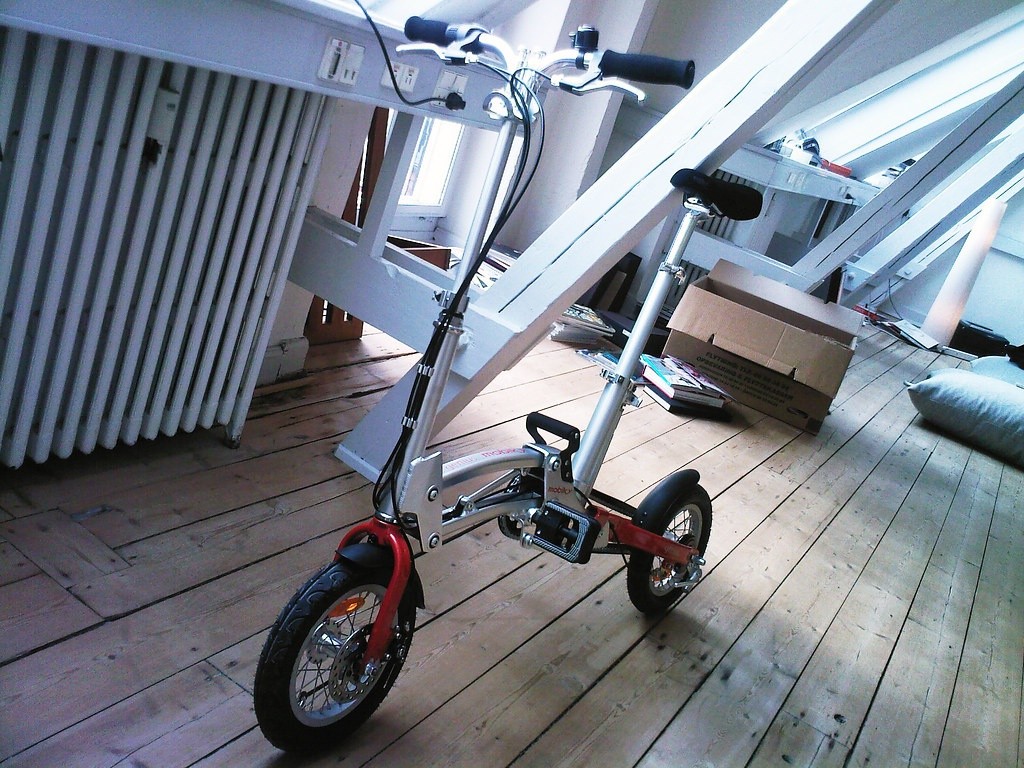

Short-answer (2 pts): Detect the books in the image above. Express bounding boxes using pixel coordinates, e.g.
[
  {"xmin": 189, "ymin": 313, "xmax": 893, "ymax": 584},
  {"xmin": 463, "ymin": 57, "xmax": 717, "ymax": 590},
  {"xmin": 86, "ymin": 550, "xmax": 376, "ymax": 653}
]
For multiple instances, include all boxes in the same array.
[
  {"xmin": 881, "ymin": 319, "xmax": 940, "ymax": 351},
  {"xmin": 576, "ymin": 348, "xmax": 743, "ymax": 423},
  {"xmin": 450, "ymin": 242, "xmax": 522, "ymax": 290},
  {"xmin": 547, "ymin": 303, "xmax": 616, "ymax": 344}
]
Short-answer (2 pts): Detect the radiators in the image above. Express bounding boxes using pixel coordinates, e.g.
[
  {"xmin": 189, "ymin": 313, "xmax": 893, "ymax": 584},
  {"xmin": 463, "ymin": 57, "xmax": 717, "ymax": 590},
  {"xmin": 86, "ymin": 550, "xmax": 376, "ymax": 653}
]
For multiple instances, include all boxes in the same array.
[{"xmin": 0, "ymin": 27, "xmax": 340, "ymax": 477}]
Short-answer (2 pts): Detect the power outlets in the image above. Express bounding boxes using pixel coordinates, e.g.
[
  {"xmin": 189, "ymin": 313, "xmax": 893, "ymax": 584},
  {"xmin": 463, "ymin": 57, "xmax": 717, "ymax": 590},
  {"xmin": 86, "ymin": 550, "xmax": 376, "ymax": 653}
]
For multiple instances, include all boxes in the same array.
[
  {"xmin": 429, "ymin": 68, "xmax": 470, "ymax": 111},
  {"xmin": 381, "ymin": 61, "xmax": 403, "ymax": 90},
  {"xmin": 401, "ymin": 64, "xmax": 420, "ymax": 92},
  {"xmin": 341, "ymin": 43, "xmax": 366, "ymax": 87},
  {"xmin": 317, "ymin": 36, "xmax": 348, "ymax": 88}
]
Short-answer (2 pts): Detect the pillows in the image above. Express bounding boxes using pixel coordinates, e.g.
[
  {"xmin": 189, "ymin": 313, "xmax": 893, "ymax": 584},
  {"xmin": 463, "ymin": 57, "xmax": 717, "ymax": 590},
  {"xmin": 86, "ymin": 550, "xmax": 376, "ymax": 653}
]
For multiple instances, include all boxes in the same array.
[{"xmin": 909, "ymin": 368, "xmax": 1024, "ymax": 459}]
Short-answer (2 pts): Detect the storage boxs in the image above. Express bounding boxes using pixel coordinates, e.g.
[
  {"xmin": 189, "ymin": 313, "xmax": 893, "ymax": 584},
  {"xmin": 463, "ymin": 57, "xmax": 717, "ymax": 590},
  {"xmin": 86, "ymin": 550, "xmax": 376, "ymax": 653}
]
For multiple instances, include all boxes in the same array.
[
  {"xmin": 663, "ymin": 258, "xmax": 865, "ymax": 436},
  {"xmin": 821, "ymin": 159, "xmax": 852, "ymax": 177},
  {"xmin": 781, "ymin": 143, "xmax": 813, "ymax": 164}
]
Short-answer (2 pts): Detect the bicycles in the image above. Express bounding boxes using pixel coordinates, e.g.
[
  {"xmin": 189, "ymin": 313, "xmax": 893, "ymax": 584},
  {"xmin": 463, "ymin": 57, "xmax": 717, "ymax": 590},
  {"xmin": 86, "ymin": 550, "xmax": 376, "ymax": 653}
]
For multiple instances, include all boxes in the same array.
[{"xmin": 251, "ymin": 18, "xmax": 763, "ymax": 759}]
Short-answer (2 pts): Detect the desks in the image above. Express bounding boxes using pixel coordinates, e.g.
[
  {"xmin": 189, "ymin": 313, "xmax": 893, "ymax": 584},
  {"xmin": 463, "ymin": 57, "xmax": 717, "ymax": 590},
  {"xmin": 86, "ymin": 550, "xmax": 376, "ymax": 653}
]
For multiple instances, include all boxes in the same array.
[{"xmin": 634, "ymin": 144, "xmax": 880, "ymax": 331}]
